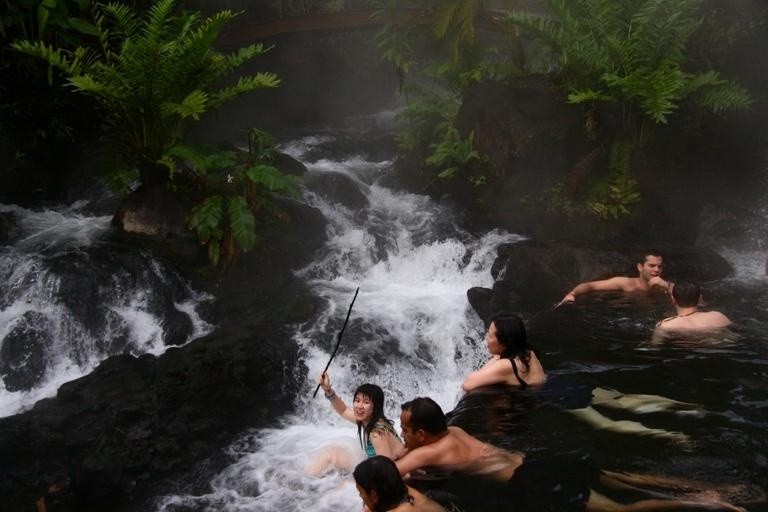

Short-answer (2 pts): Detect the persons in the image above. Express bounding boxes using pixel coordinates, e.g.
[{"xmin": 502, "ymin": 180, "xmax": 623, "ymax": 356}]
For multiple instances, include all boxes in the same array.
[
  {"xmin": 649, "ymin": 280, "xmax": 745, "ymax": 349},
  {"xmin": 551, "ymin": 249, "xmax": 707, "ymax": 313},
  {"xmin": 297, "ymin": 371, "xmax": 411, "ymax": 493},
  {"xmin": 352, "ymin": 455, "xmax": 449, "ymax": 512},
  {"xmin": 393, "ymin": 397, "xmax": 765, "ymax": 512},
  {"xmin": 460, "ymin": 311, "xmax": 708, "ymax": 457}
]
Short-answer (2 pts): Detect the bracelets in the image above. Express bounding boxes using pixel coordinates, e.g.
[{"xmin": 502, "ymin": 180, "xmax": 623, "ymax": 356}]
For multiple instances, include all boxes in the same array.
[
  {"xmin": 324, "ymin": 388, "xmax": 336, "ymax": 400},
  {"xmin": 665, "ymin": 280, "xmax": 670, "ymax": 290}
]
